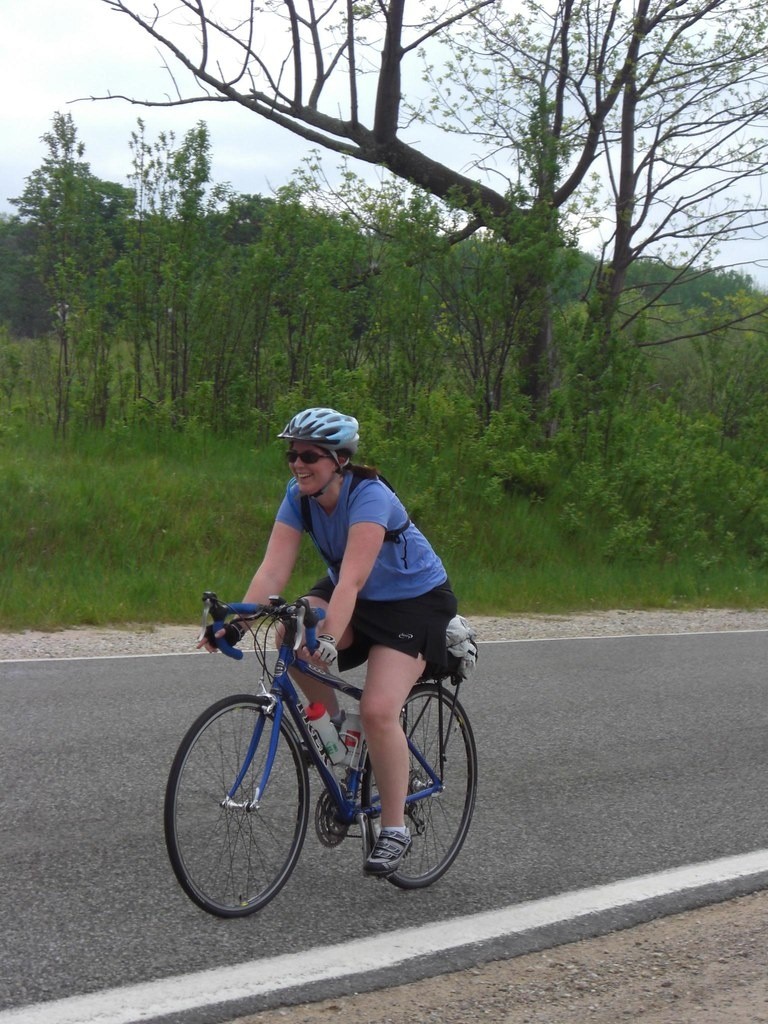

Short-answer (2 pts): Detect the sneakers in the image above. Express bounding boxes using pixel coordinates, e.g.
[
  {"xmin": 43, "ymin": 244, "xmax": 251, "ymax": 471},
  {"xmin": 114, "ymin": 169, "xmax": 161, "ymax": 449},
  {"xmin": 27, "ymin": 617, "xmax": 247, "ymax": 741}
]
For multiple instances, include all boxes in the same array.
[
  {"xmin": 363, "ymin": 826, "xmax": 411, "ymax": 879},
  {"xmin": 299, "ymin": 709, "xmax": 347, "ymax": 760}
]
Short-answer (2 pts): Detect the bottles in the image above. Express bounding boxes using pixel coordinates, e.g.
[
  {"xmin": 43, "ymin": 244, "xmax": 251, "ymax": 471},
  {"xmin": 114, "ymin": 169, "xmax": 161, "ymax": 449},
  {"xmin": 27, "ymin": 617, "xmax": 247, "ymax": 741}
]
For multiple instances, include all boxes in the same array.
[
  {"xmin": 305, "ymin": 701, "xmax": 347, "ymax": 764},
  {"xmin": 339, "ymin": 709, "xmax": 365, "ymax": 766}
]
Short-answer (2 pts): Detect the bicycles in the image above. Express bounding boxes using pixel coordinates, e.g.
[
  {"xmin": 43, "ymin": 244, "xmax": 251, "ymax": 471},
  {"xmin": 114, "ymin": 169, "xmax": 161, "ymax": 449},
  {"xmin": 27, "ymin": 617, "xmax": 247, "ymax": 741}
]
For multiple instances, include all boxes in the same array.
[{"xmin": 164, "ymin": 592, "xmax": 480, "ymax": 919}]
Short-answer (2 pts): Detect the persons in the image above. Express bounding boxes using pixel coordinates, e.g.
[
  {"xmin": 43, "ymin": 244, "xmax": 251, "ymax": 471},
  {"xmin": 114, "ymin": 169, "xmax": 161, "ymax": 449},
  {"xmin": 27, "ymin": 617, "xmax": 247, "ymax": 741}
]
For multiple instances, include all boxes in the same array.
[{"xmin": 197, "ymin": 407, "xmax": 456, "ymax": 877}]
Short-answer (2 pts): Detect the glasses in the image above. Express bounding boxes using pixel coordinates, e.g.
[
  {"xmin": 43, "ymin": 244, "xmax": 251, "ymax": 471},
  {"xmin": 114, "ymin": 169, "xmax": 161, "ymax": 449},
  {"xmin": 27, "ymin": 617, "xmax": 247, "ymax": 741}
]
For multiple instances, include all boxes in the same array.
[{"xmin": 284, "ymin": 450, "xmax": 321, "ymax": 464}]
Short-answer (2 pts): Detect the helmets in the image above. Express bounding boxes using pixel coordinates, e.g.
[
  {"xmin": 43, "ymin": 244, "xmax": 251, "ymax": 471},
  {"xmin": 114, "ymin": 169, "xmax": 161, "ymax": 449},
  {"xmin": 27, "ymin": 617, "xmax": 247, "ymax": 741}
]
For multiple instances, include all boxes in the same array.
[{"xmin": 276, "ymin": 407, "xmax": 360, "ymax": 456}]
